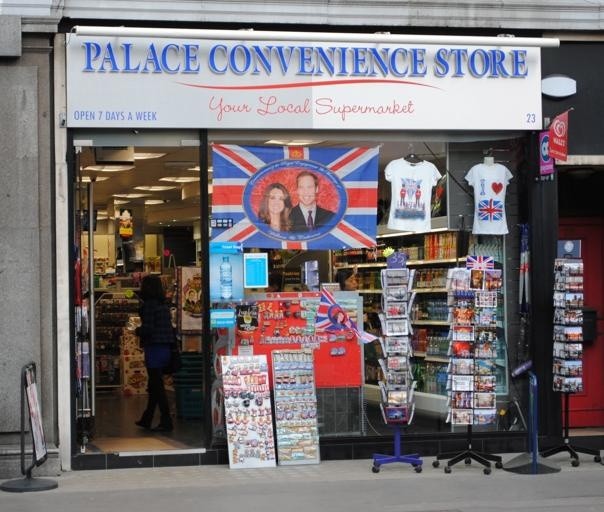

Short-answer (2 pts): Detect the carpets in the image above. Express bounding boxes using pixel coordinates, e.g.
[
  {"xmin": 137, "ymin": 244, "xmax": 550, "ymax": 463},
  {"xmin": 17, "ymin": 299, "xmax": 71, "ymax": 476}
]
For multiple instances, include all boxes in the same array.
[{"xmin": 89, "ymin": 431, "xmax": 207, "ymax": 457}]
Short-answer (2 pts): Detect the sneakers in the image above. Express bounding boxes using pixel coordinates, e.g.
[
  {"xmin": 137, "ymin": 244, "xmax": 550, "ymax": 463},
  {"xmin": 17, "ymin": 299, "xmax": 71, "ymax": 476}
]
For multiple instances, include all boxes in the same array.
[
  {"xmin": 155, "ymin": 424, "xmax": 173, "ymax": 432},
  {"xmin": 135, "ymin": 421, "xmax": 152, "ymax": 427}
]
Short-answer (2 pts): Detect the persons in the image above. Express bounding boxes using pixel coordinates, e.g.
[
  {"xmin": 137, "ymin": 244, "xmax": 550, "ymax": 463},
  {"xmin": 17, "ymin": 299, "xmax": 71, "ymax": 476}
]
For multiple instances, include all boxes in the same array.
[
  {"xmin": 125, "ymin": 274, "xmax": 180, "ymax": 433},
  {"xmin": 286, "ymin": 171, "xmax": 337, "ymax": 235},
  {"xmin": 257, "ymin": 181, "xmax": 291, "ymax": 233},
  {"xmin": 185, "ymin": 289, "xmax": 197, "ymax": 304},
  {"xmin": 333, "ymin": 311, "xmax": 344, "ymax": 323}
]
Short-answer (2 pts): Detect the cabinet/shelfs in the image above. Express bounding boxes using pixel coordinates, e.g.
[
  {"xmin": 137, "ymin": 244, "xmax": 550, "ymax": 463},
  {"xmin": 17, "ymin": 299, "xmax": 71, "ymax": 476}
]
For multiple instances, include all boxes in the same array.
[{"xmin": 87, "ymin": 217, "xmax": 523, "ymax": 423}]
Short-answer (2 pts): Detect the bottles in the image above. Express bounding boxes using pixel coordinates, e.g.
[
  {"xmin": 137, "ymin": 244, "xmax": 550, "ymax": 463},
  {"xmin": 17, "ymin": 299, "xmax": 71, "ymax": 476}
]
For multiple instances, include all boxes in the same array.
[
  {"xmin": 411, "ymin": 231, "xmax": 473, "ymax": 398},
  {"xmin": 219, "ymin": 256, "xmax": 232, "ymax": 299}
]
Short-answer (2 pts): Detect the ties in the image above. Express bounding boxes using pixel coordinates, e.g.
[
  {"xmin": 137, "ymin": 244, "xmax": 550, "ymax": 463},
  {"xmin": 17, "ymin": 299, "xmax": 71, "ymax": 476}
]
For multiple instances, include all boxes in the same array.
[{"xmin": 308, "ymin": 211, "xmax": 313, "ymax": 231}]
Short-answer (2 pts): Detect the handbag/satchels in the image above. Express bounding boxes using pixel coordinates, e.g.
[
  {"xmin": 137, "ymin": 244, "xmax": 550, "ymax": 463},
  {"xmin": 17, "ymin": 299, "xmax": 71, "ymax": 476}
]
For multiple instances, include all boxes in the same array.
[{"xmin": 146, "ymin": 342, "xmax": 178, "ymax": 375}]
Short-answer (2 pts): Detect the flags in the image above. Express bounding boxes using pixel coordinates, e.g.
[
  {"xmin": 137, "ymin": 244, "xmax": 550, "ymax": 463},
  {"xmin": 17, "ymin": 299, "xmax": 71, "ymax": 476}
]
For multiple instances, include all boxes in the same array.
[
  {"xmin": 312, "ymin": 288, "xmax": 359, "ymax": 336},
  {"xmin": 463, "ymin": 254, "xmax": 495, "ymax": 270},
  {"xmin": 208, "ymin": 142, "xmax": 383, "ymax": 252},
  {"xmin": 546, "ymin": 113, "xmax": 570, "ymax": 163},
  {"xmin": 476, "ymin": 199, "xmax": 504, "ymax": 223}
]
